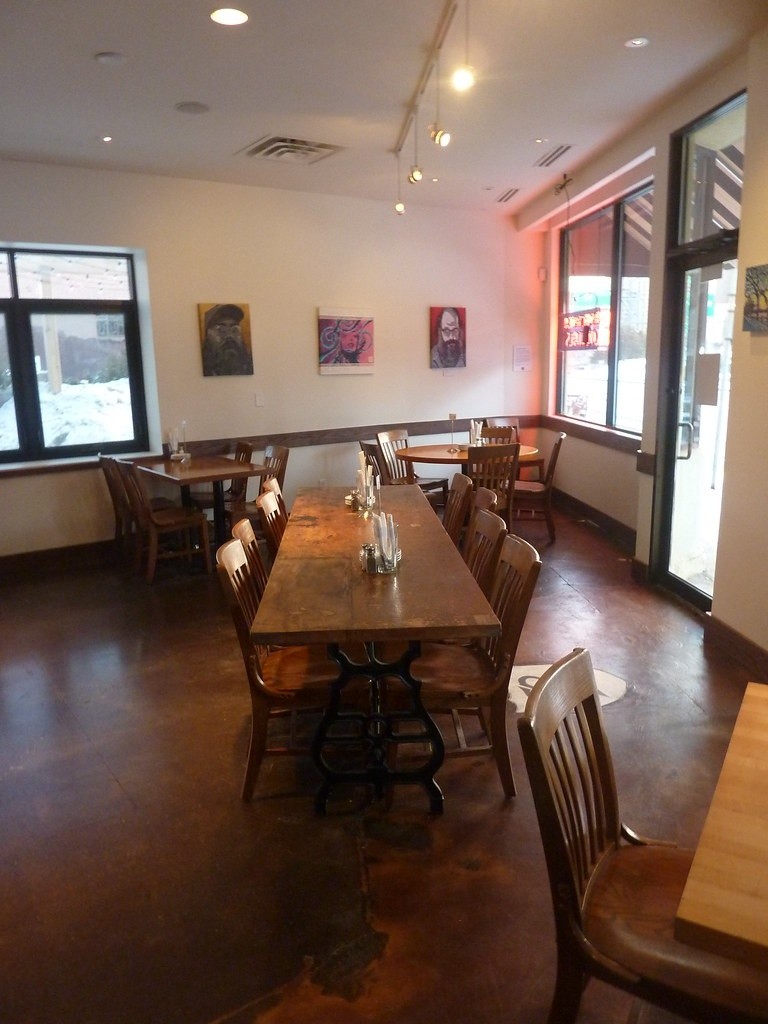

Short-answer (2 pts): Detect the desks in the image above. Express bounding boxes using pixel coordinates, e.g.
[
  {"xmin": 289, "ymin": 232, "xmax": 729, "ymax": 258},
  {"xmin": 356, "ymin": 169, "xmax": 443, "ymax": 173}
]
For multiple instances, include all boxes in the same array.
[
  {"xmin": 124, "ymin": 453, "xmax": 274, "ymax": 576},
  {"xmin": 395, "ymin": 443, "xmax": 539, "ymax": 527},
  {"xmin": 673, "ymin": 684, "xmax": 768, "ymax": 970},
  {"xmin": 249, "ymin": 484, "xmax": 502, "ymax": 819}
]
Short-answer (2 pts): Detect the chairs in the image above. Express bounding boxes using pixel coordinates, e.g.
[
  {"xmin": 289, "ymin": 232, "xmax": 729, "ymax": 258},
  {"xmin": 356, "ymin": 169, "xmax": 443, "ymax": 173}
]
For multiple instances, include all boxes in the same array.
[
  {"xmin": 190, "ymin": 440, "xmax": 254, "ymax": 548},
  {"xmin": 467, "ymin": 442, "xmax": 521, "ymax": 534},
  {"xmin": 500, "ymin": 432, "xmax": 566, "ymax": 543},
  {"xmin": 442, "ymin": 473, "xmax": 474, "ymax": 547},
  {"xmin": 224, "ymin": 445, "xmax": 289, "ymax": 540},
  {"xmin": 231, "ymin": 518, "xmax": 285, "ymax": 652},
  {"xmin": 255, "ymin": 490, "xmax": 284, "ymax": 557},
  {"xmin": 376, "ymin": 429, "xmax": 448, "ymax": 507},
  {"xmin": 263, "ymin": 476, "xmax": 288, "ymax": 524},
  {"xmin": 113, "ymin": 457, "xmax": 212, "ymax": 586},
  {"xmin": 215, "ymin": 537, "xmax": 375, "ymax": 802},
  {"xmin": 382, "ymin": 534, "xmax": 542, "ymax": 801},
  {"xmin": 460, "ymin": 486, "xmax": 497, "ymax": 564},
  {"xmin": 385, "ymin": 507, "xmax": 508, "ymax": 751},
  {"xmin": 516, "ymin": 647, "xmax": 768, "ymax": 1024},
  {"xmin": 358, "ymin": 440, "xmax": 438, "ymax": 507},
  {"xmin": 96, "ymin": 451, "xmax": 184, "ymax": 564},
  {"xmin": 486, "ymin": 417, "xmax": 544, "ymax": 484}
]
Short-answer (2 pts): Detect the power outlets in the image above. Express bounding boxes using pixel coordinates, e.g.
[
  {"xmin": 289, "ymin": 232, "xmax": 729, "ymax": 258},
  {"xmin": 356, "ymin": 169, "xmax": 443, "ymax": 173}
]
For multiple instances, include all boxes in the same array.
[
  {"xmin": 319, "ymin": 478, "xmax": 327, "ymax": 487},
  {"xmin": 254, "ymin": 392, "xmax": 266, "ymax": 407}
]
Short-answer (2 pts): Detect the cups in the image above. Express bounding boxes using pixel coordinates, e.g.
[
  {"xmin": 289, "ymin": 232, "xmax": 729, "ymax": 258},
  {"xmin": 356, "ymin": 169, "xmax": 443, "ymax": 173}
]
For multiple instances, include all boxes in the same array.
[{"xmin": 378, "ymin": 549, "xmax": 398, "ymax": 574}]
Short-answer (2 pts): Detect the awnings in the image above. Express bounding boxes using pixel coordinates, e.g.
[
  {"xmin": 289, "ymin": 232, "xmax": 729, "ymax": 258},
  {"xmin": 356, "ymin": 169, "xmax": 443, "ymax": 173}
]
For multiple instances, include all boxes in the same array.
[{"xmin": 601, "ymin": 139, "xmax": 745, "ymax": 273}]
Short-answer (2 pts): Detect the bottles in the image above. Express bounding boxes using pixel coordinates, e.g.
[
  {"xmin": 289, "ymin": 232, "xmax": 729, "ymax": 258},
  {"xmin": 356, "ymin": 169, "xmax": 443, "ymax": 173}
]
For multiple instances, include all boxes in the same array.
[
  {"xmin": 359, "ymin": 544, "xmax": 367, "ymax": 570},
  {"xmin": 366, "ymin": 549, "xmax": 376, "ymax": 575},
  {"xmin": 476, "ymin": 437, "xmax": 482, "ymax": 447},
  {"xmin": 351, "ymin": 492, "xmax": 359, "ymax": 511}
]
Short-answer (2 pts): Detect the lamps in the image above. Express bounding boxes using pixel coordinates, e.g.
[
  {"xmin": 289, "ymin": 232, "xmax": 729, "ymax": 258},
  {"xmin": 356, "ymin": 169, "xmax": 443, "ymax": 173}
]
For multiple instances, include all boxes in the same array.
[
  {"xmin": 429, "ymin": 52, "xmax": 450, "ymax": 147},
  {"xmin": 394, "ymin": 152, "xmax": 406, "ymax": 215},
  {"xmin": 408, "ymin": 115, "xmax": 422, "ymax": 184}
]
